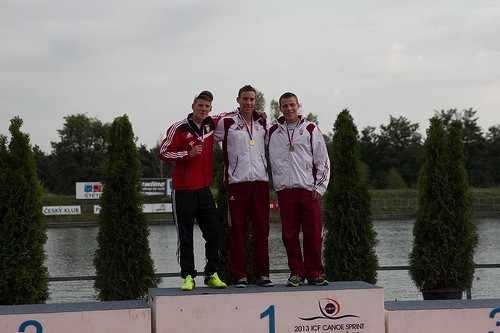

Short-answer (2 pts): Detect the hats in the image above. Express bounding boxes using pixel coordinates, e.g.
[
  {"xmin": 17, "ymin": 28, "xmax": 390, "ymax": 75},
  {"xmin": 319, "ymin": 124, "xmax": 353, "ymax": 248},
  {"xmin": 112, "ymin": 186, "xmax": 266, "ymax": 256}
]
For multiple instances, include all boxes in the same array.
[{"xmin": 195, "ymin": 88, "xmax": 214, "ymax": 102}]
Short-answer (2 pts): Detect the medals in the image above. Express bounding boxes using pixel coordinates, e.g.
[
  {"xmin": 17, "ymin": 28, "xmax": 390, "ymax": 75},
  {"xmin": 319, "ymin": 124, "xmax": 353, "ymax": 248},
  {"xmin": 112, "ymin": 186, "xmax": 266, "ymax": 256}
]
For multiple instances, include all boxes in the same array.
[
  {"xmin": 199, "ymin": 141, "xmax": 205, "ymax": 147},
  {"xmin": 249, "ymin": 140, "xmax": 255, "ymax": 145},
  {"xmin": 288, "ymin": 146, "xmax": 294, "ymax": 153}
]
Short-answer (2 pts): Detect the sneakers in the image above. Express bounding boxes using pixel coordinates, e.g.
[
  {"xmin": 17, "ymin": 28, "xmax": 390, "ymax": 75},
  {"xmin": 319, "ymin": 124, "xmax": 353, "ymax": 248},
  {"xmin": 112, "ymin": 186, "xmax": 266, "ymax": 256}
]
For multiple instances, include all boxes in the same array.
[
  {"xmin": 204, "ymin": 272, "xmax": 227, "ymax": 288},
  {"xmin": 180, "ymin": 275, "xmax": 195, "ymax": 290},
  {"xmin": 235, "ymin": 277, "xmax": 248, "ymax": 288},
  {"xmin": 307, "ymin": 277, "xmax": 329, "ymax": 286},
  {"xmin": 257, "ymin": 276, "xmax": 274, "ymax": 287},
  {"xmin": 287, "ymin": 275, "xmax": 305, "ymax": 287}
]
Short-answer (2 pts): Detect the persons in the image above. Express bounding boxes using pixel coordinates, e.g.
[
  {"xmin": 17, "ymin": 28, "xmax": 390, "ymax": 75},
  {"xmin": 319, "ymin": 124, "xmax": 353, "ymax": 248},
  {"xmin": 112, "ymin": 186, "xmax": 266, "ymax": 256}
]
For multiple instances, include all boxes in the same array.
[
  {"xmin": 157, "ymin": 90, "xmax": 266, "ymax": 290},
  {"xmin": 267, "ymin": 92, "xmax": 331, "ymax": 287},
  {"xmin": 213, "ymin": 85, "xmax": 271, "ymax": 289}
]
